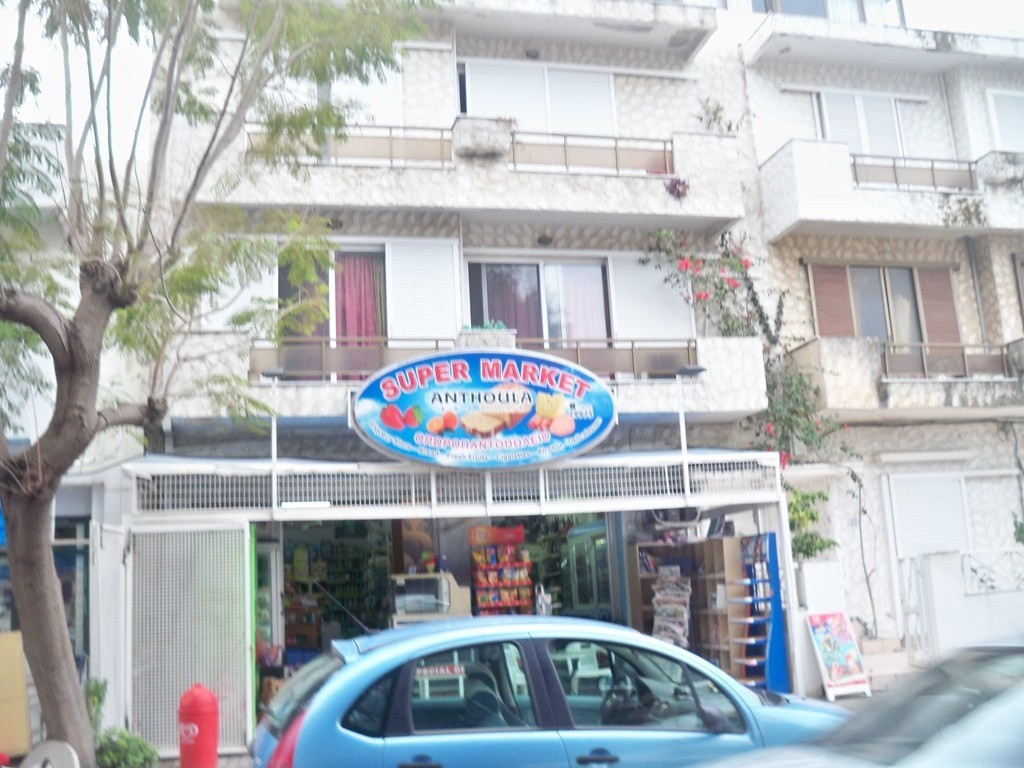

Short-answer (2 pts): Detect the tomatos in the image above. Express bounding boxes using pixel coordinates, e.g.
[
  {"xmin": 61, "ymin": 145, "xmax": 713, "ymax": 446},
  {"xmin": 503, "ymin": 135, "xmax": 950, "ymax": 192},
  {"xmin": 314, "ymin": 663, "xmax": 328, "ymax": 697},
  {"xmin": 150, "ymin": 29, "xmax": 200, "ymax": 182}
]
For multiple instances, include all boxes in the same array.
[
  {"xmin": 380, "ymin": 406, "xmax": 404, "ymax": 430},
  {"xmin": 404, "ymin": 408, "xmax": 418, "ymax": 427}
]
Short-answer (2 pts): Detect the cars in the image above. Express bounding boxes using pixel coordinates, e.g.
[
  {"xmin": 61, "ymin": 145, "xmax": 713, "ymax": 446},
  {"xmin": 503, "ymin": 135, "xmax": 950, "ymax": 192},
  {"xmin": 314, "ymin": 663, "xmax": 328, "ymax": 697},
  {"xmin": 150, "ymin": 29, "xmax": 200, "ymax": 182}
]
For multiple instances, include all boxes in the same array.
[
  {"xmin": 680, "ymin": 639, "xmax": 1024, "ymax": 768},
  {"xmin": 241, "ymin": 615, "xmax": 854, "ymax": 768}
]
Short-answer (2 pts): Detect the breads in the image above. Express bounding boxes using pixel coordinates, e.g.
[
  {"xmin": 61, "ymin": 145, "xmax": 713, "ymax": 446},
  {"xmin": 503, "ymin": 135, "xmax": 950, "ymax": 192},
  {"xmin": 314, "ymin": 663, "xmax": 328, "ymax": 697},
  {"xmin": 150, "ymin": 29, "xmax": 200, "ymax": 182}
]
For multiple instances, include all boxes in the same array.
[
  {"xmin": 460, "ymin": 412, "xmax": 504, "ymax": 437},
  {"xmin": 482, "ymin": 384, "xmax": 532, "ymax": 428}
]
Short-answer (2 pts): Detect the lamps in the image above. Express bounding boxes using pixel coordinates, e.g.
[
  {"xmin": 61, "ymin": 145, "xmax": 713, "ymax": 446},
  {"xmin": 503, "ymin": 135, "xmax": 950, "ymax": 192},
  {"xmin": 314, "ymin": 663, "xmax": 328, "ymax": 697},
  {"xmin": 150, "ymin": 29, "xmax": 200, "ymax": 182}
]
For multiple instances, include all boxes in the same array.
[
  {"xmin": 261, "ymin": 367, "xmax": 286, "ymax": 378},
  {"xmin": 675, "ymin": 366, "xmax": 706, "ymax": 379}
]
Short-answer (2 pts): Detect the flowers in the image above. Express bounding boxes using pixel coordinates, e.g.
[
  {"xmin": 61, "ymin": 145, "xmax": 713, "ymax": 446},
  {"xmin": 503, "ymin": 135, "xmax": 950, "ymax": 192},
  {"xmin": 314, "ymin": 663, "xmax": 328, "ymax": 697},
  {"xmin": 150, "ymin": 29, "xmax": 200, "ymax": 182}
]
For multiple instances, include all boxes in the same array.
[{"xmin": 661, "ymin": 177, "xmax": 691, "ymax": 199}]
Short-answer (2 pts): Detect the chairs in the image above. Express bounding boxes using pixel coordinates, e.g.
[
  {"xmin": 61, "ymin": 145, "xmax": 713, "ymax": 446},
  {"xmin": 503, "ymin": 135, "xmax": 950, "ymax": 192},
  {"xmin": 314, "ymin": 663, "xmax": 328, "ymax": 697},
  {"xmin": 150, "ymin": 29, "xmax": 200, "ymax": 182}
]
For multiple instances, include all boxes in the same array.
[{"xmin": 464, "ymin": 661, "xmax": 533, "ymax": 728}]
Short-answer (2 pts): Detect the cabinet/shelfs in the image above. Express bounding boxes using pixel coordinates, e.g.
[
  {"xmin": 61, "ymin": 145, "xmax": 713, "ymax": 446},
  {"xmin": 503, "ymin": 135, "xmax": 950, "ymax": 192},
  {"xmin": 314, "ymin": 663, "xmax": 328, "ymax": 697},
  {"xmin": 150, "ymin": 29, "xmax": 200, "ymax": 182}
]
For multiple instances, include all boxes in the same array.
[
  {"xmin": 468, "ymin": 524, "xmax": 534, "ymax": 616},
  {"xmin": 625, "ymin": 531, "xmax": 792, "ymax": 696}
]
